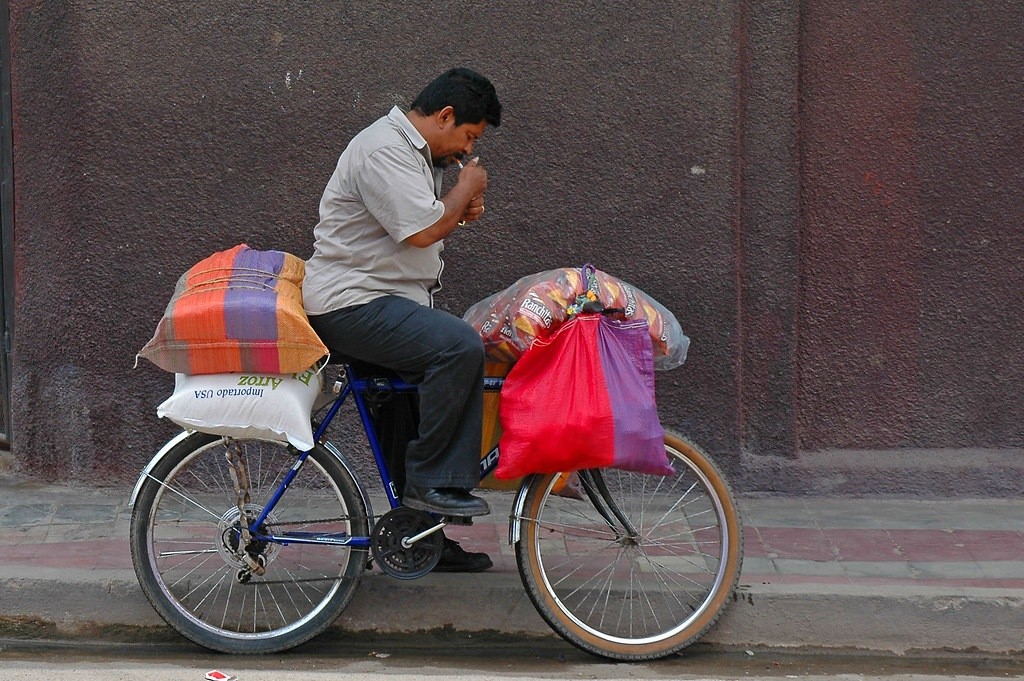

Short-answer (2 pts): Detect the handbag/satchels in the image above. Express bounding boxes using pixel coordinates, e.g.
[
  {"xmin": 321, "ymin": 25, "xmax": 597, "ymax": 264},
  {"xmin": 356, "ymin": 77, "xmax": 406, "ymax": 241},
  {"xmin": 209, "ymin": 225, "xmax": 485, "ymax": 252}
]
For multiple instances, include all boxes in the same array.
[
  {"xmin": 493, "ymin": 264, "xmax": 674, "ymax": 479},
  {"xmin": 475, "ymin": 352, "xmax": 585, "ymax": 502}
]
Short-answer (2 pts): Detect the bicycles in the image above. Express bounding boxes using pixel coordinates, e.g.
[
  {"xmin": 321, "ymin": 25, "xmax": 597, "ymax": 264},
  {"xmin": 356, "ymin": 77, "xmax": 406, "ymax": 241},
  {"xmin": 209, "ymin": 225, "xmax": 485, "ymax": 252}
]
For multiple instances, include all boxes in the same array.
[{"xmin": 125, "ymin": 290, "xmax": 746, "ymax": 662}]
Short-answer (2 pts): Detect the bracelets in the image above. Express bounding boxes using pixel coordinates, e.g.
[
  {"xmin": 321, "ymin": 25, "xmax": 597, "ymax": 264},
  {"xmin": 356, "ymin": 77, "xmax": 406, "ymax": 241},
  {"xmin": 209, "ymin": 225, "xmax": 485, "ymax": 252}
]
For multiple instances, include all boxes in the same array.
[{"xmin": 458, "ymin": 221, "xmax": 465, "ymax": 226}]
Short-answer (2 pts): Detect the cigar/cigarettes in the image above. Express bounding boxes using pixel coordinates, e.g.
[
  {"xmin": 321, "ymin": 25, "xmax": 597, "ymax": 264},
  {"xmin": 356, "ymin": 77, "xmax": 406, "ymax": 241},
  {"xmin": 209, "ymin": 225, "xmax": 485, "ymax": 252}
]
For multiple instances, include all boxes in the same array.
[{"xmin": 457, "ymin": 159, "xmax": 464, "ymax": 169}]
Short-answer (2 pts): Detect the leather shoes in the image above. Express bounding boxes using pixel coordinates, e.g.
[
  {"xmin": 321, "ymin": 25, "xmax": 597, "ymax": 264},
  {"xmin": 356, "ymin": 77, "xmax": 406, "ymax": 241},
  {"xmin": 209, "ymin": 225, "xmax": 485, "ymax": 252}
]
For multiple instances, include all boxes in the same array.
[
  {"xmin": 431, "ymin": 538, "xmax": 492, "ymax": 573},
  {"xmin": 402, "ymin": 485, "xmax": 490, "ymax": 516}
]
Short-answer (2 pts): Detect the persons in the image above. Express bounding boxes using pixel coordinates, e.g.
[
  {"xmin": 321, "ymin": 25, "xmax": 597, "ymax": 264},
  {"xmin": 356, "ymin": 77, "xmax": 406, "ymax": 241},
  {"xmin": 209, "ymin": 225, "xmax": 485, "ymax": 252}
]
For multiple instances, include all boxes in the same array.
[{"xmin": 303, "ymin": 68, "xmax": 501, "ymax": 572}]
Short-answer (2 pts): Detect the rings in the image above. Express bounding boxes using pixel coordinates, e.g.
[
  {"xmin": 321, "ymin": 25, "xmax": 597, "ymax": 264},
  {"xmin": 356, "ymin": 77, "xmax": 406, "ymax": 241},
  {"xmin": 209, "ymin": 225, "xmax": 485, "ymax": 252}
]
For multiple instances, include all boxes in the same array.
[{"xmin": 480, "ymin": 205, "xmax": 484, "ymax": 213}]
